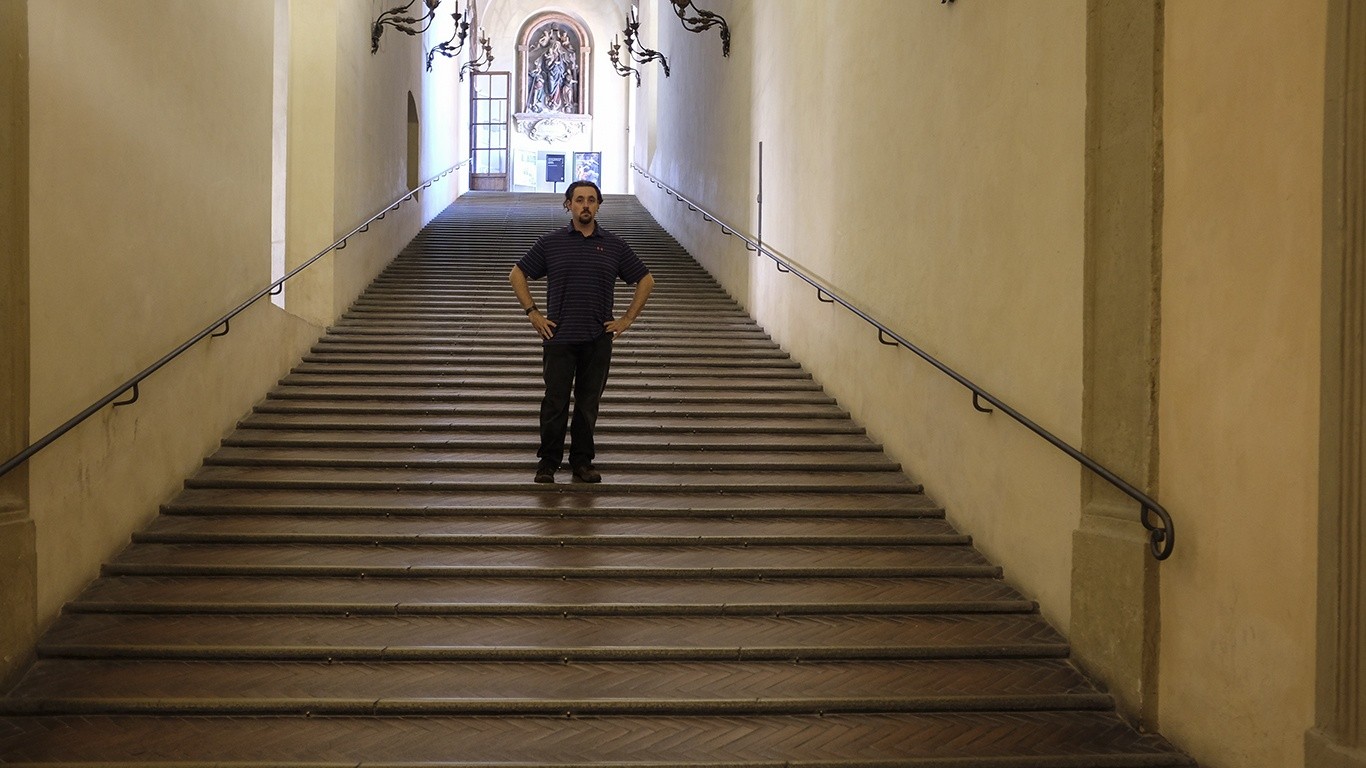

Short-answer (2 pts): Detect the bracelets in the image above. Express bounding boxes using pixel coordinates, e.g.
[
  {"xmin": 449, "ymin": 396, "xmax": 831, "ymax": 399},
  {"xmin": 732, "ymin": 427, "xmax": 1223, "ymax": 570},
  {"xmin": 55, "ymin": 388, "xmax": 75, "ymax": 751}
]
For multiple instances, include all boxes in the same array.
[{"xmin": 526, "ymin": 305, "xmax": 538, "ymax": 315}]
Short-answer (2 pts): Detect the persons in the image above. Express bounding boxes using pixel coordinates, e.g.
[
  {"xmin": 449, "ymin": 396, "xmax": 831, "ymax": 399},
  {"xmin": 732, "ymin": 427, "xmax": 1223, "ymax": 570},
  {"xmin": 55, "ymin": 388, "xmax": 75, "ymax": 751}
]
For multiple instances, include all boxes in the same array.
[{"xmin": 507, "ymin": 180, "xmax": 655, "ymax": 484}]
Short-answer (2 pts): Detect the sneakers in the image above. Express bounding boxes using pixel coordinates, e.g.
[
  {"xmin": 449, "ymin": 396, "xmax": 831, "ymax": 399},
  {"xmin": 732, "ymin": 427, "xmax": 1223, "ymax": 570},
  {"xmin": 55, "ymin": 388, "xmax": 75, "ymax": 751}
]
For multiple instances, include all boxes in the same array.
[
  {"xmin": 534, "ymin": 460, "xmax": 556, "ymax": 482},
  {"xmin": 573, "ymin": 464, "xmax": 602, "ymax": 482}
]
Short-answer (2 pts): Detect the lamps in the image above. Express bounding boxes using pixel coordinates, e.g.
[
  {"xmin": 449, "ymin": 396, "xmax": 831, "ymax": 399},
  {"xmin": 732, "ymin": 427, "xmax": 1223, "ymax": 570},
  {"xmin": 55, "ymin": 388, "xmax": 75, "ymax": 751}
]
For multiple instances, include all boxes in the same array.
[
  {"xmin": 624, "ymin": 4, "xmax": 669, "ymax": 78},
  {"xmin": 371, "ymin": 0, "xmax": 441, "ymax": 54},
  {"xmin": 608, "ymin": 34, "xmax": 641, "ymax": 88},
  {"xmin": 425, "ymin": 0, "xmax": 470, "ymax": 72},
  {"xmin": 459, "ymin": 29, "xmax": 494, "ymax": 82},
  {"xmin": 670, "ymin": 0, "xmax": 731, "ymax": 57}
]
